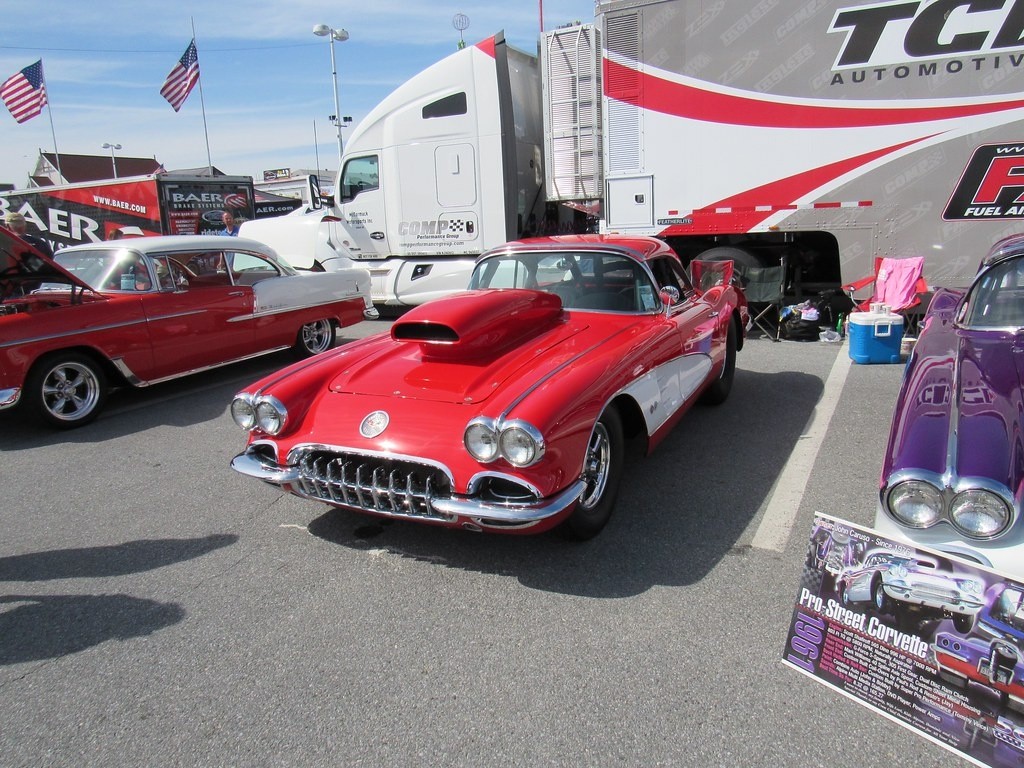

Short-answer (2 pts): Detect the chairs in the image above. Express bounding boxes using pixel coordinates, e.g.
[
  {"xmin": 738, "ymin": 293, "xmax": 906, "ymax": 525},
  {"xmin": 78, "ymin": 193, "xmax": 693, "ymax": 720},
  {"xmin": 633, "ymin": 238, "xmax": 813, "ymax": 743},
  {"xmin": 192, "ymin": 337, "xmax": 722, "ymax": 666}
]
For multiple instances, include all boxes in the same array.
[
  {"xmin": 690, "ymin": 259, "xmax": 734, "ymax": 296},
  {"xmin": 742, "ymin": 256, "xmax": 788, "ymax": 342},
  {"xmin": 136, "ymin": 270, "xmax": 177, "ymax": 294},
  {"xmin": 575, "ymin": 292, "xmax": 632, "ymax": 311},
  {"xmin": 842, "ymin": 256, "xmax": 926, "ymax": 338}
]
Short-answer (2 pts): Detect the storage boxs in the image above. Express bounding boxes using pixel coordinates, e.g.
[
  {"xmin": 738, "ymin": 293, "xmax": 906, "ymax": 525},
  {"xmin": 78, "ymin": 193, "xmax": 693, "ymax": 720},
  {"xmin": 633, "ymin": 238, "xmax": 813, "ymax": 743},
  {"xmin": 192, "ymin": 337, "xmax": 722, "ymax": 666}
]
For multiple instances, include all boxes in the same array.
[{"xmin": 848, "ymin": 302, "xmax": 904, "ymax": 364}]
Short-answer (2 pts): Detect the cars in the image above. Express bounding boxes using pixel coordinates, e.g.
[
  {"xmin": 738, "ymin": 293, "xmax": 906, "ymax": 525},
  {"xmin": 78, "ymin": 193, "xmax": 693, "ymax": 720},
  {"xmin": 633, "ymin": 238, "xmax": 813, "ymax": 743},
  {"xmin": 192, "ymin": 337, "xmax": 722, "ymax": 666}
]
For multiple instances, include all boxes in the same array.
[
  {"xmin": 229, "ymin": 233, "xmax": 749, "ymax": 542},
  {"xmin": 0, "ymin": 225, "xmax": 380, "ymax": 432},
  {"xmin": 874, "ymin": 234, "xmax": 1024, "ymax": 584},
  {"xmin": 811, "ymin": 527, "xmax": 1024, "ymax": 716}
]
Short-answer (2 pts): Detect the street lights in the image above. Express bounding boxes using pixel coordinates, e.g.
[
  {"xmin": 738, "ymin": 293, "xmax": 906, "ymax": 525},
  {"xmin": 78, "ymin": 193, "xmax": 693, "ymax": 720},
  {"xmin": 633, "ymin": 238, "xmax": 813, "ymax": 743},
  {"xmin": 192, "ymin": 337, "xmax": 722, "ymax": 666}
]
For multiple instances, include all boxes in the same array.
[
  {"xmin": 313, "ymin": 24, "xmax": 352, "ymax": 163},
  {"xmin": 102, "ymin": 143, "xmax": 122, "ymax": 179}
]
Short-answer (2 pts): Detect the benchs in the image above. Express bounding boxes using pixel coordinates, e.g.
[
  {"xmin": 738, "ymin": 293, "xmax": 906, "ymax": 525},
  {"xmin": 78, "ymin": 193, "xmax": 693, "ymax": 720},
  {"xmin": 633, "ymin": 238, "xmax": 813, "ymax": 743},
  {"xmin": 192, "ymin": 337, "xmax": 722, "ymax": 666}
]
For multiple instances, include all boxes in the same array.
[{"xmin": 188, "ymin": 272, "xmax": 274, "ymax": 286}]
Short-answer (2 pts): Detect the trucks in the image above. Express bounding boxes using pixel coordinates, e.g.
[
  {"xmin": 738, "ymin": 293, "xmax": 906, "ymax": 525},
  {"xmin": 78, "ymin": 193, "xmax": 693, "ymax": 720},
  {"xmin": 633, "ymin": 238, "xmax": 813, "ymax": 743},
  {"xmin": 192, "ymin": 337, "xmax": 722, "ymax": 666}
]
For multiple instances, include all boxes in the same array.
[{"xmin": 237, "ymin": 0, "xmax": 1024, "ymax": 321}]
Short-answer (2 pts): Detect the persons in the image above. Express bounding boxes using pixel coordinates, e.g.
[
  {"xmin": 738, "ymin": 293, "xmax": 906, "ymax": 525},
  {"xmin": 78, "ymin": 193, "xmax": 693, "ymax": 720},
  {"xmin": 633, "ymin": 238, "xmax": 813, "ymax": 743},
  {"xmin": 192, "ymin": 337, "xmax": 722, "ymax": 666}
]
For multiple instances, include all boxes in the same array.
[
  {"xmin": 100, "ymin": 228, "xmax": 131, "ymax": 289},
  {"xmin": 216, "ymin": 211, "xmax": 240, "ymax": 276},
  {"xmin": 2, "ymin": 211, "xmax": 56, "ymax": 264}
]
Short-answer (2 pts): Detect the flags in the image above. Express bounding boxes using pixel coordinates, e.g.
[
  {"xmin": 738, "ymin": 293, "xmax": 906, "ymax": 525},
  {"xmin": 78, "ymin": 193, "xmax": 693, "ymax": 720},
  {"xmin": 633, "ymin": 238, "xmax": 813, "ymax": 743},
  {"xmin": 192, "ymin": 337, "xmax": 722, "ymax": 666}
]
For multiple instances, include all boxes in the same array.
[
  {"xmin": 0, "ymin": 59, "xmax": 52, "ymax": 125},
  {"xmin": 153, "ymin": 37, "xmax": 210, "ymax": 119}
]
